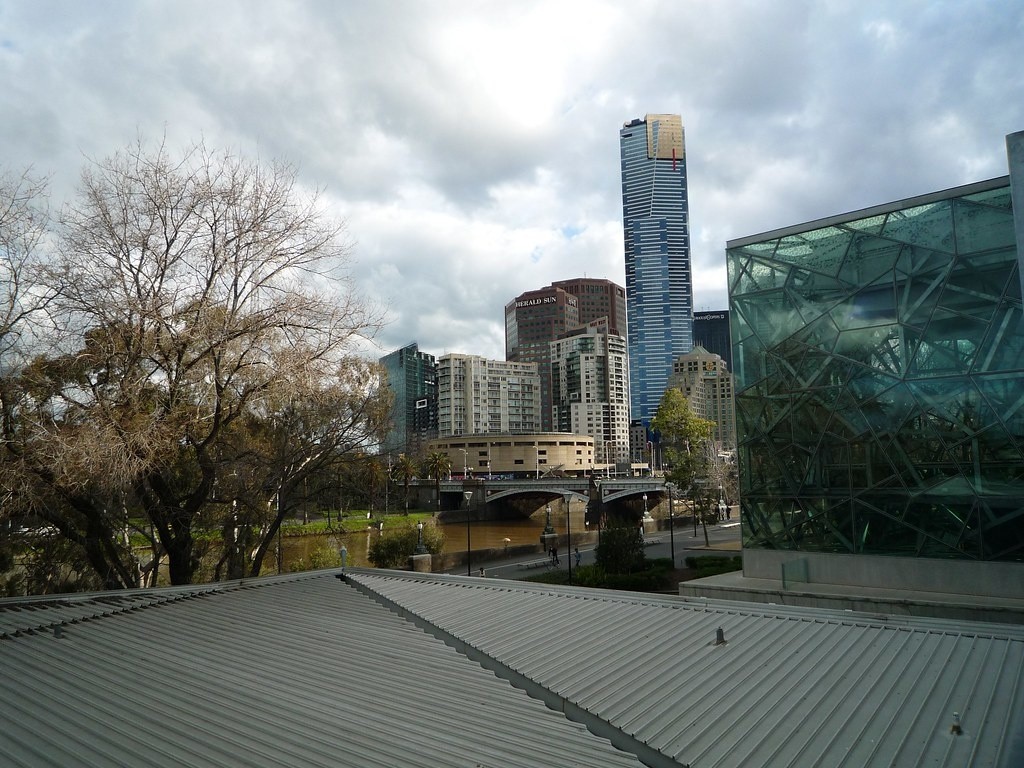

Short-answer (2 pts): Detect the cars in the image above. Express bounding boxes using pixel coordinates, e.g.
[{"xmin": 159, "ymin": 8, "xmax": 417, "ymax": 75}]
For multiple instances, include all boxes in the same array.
[
  {"xmin": 594, "ymin": 475, "xmax": 602, "ymax": 480},
  {"xmin": 411, "ymin": 476, "xmax": 417, "ymax": 482}
]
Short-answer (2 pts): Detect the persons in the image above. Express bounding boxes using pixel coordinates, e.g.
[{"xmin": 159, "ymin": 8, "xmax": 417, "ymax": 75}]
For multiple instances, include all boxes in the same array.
[
  {"xmin": 479, "ymin": 567, "xmax": 487, "ymax": 578},
  {"xmin": 573, "ymin": 548, "xmax": 581, "ymax": 568},
  {"xmin": 547, "ymin": 545, "xmax": 561, "ymax": 567},
  {"xmin": 714, "ymin": 503, "xmax": 732, "ymax": 522}
]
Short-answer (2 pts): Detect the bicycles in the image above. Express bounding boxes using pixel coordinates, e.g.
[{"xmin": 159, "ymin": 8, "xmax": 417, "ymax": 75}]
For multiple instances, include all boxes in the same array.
[{"xmin": 547, "ymin": 556, "xmax": 562, "ymax": 570}]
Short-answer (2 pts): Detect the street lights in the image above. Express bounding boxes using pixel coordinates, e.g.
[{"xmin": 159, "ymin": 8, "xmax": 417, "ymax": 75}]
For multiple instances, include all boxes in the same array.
[
  {"xmin": 488, "ymin": 442, "xmax": 495, "ymax": 480},
  {"xmin": 648, "ymin": 441, "xmax": 653, "ymax": 477},
  {"xmin": 533, "ymin": 446, "xmax": 539, "ymax": 479},
  {"xmin": 606, "ymin": 440, "xmax": 612, "ymax": 478},
  {"xmin": 465, "ymin": 490, "xmax": 473, "ymax": 576},
  {"xmin": 563, "ymin": 490, "xmax": 574, "ymax": 585},
  {"xmin": 459, "ymin": 449, "xmax": 466, "ymax": 479}
]
